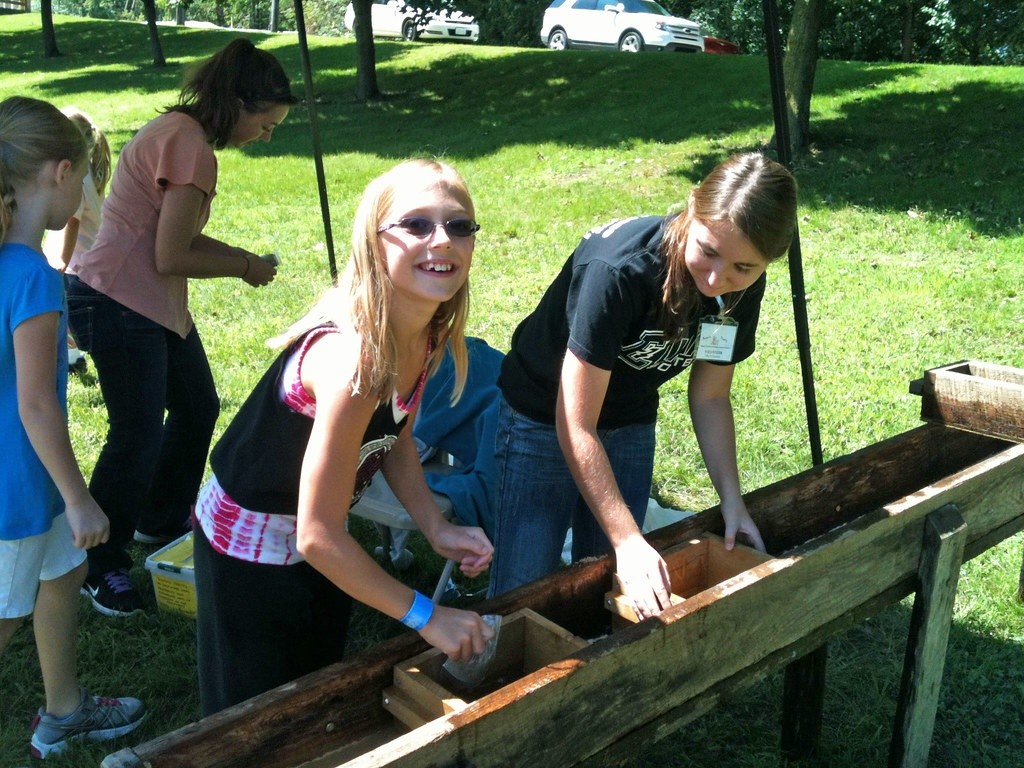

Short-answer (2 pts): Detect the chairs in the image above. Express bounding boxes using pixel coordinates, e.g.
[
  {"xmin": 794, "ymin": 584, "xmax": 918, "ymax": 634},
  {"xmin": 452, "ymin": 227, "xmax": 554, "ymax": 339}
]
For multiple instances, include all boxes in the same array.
[{"xmin": 345, "ymin": 332, "xmax": 514, "ymax": 609}]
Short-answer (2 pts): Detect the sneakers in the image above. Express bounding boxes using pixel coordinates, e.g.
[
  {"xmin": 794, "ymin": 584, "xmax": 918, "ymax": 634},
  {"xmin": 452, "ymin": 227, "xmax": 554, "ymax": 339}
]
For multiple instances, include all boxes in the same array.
[
  {"xmin": 134, "ymin": 515, "xmax": 195, "ymax": 542},
  {"xmin": 30, "ymin": 687, "xmax": 147, "ymax": 759},
  {"xmin": 80, "ymin": 565, "xmax": 149, "ymax": 616}
]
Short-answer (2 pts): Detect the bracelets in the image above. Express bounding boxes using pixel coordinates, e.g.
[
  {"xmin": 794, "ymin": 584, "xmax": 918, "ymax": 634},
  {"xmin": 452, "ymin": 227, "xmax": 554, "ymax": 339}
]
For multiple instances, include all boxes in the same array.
[
  {"xmin": 242, "ymin": 255, "xmax": 254, "ymax": 281},
  {"xmin": 399, "ymin": 590, "xmax": 436, "ymax": 631}
]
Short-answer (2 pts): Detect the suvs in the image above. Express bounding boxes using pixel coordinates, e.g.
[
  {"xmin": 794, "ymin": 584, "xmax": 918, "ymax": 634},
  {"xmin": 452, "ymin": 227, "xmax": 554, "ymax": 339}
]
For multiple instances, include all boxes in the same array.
[{"xmin": 540, "ymin": 0, "xmax": 704, "ymax": 53}]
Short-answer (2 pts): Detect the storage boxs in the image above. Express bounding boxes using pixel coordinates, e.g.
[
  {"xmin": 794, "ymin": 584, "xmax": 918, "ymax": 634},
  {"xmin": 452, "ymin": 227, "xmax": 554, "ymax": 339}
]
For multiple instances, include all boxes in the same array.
[{"xmin": 143, "ymin": 532, "xmax": 200, "ymax": 620}]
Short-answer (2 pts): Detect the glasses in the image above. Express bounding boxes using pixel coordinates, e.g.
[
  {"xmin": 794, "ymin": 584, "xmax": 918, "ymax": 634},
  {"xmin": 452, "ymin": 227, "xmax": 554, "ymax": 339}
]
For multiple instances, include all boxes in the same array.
[{"xmin": 376, "ymin": 214, "xmax": 481, "ymax": 237}]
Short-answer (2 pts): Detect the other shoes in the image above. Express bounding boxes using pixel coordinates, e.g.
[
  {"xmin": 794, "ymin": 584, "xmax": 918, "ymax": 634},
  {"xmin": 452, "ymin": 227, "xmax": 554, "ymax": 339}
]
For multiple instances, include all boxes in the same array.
[{"xmin": 387, "ymin": 558, "xmax": 436, "ymax": 592}]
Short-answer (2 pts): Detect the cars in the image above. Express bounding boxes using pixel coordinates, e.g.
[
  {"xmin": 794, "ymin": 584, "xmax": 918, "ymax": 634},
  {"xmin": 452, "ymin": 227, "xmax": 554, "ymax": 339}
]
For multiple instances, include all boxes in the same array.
[{"xmin": 344, "ymin": 0, "xmax": 479, "ymax": 44}]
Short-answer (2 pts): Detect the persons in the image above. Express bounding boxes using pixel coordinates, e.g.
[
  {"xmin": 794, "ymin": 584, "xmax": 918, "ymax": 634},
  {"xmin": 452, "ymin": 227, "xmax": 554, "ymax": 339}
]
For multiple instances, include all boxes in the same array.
[
  {"xmin": 194, "ymin": 156, "xmax": 498, "ymax": 715},
  {"xmin": 0, "ymin": 96, "xmax": 150, "ymax": 760},
  {"xmin": 489, "ymin": 152, "xmax": 799, "ymax": 625},
  {"xmin": 66, "ymin": 38, "xmax": 300, "ymax": 621},
  {"xmin": 59, "ymin": 114, "xmax": 112, "ymax": 370}
]
